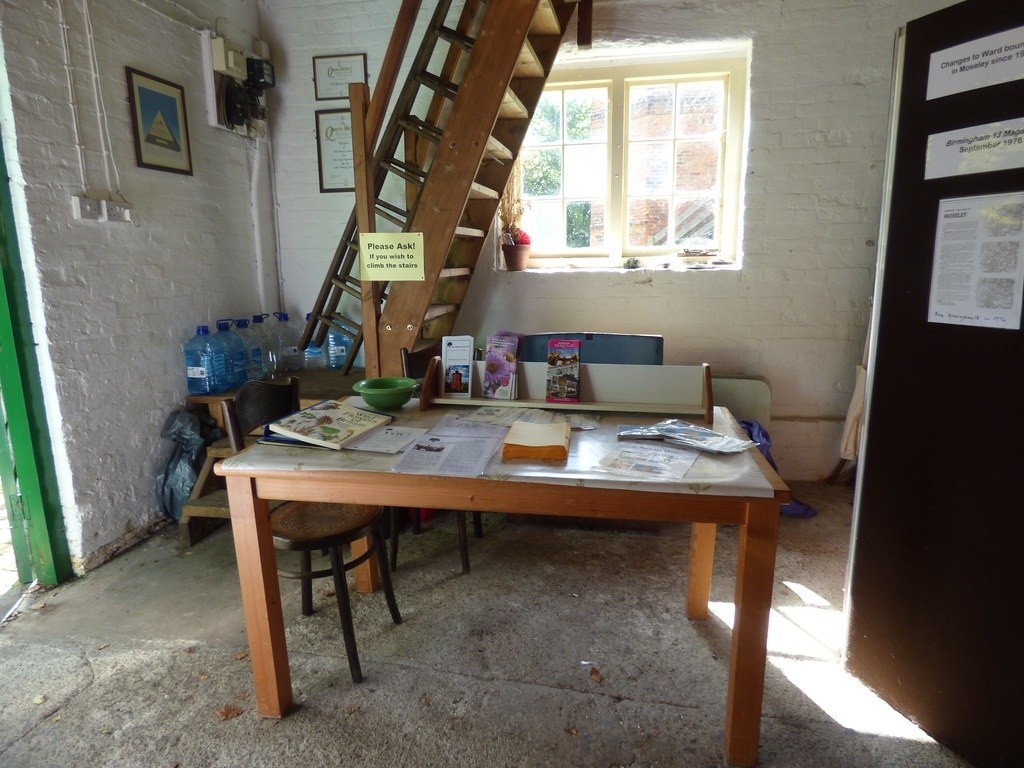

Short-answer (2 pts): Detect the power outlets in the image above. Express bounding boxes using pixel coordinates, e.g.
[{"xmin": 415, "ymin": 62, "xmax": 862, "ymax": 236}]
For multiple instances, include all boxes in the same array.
[{"xmin": 101, "ymin": 200, "xmax": 132, "ymax": 222}]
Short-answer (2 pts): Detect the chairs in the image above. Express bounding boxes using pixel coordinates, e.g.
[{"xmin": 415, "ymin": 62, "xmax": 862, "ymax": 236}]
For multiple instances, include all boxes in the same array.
[
  {"xmin": 222, "ymin": 376, "xmax": 402, "ymax": 683},
  {"xmin": 390, "ymin": 338, "xmax": 484, "ymax": 575}
]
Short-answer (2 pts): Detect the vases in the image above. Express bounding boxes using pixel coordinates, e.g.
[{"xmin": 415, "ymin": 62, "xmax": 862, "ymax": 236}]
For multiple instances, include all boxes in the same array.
[{"xmin": 502, "ymin": 244, "xmax": 531, "ymax": 270}]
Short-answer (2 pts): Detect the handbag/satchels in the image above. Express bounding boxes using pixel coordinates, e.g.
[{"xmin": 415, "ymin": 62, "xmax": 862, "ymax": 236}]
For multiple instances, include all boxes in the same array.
[{"xmin": 840, "ymin": 303, "xmax": 868, "ymax": 460}]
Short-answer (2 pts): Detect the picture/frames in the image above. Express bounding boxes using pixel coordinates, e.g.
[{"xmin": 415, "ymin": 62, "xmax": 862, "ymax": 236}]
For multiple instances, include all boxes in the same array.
[
  {"xmin": 315, "ymin": 108, "xmax": 355, "ymax": 193},
  {"xmin": 125, "ymin": 66, "xmax": 193, "ymax": 176},
  {"xmin": 313, "ymin": 53, "xmax": 368, "ymax": 101}
]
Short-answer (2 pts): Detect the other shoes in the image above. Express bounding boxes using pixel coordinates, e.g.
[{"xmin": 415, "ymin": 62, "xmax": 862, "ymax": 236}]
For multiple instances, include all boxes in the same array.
[{"xmin": 779, "ymin": 499, "xmax": 817, "ymax": 518}]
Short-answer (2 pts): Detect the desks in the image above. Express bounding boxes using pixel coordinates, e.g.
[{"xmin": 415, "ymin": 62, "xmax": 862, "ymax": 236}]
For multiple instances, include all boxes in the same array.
[{"xmin": 215, "ymin": 396, "xmax": 790, "ymax": 768}]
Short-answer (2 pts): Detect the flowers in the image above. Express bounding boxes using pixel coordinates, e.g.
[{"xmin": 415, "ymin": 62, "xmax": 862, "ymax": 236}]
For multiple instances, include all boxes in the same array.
[{"xmin": 513, "ymin": 228, "xmax": 531, "ymax": 245}]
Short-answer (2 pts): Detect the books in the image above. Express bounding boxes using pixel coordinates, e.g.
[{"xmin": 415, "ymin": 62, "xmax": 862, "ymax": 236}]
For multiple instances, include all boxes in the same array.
[
  {"xmin": 502, "ymin": 420, "xmax": 571, "ymax": 460},
  {"xmin": 271, "ymin": 399, "xmax": 392, "ymax": 451}
]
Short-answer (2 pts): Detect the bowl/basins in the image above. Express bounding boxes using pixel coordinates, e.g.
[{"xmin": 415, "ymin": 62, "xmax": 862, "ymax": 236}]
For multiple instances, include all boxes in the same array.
[{"xmin": 353, "ymin": 377, "xmax": 420, "ymax": 409}]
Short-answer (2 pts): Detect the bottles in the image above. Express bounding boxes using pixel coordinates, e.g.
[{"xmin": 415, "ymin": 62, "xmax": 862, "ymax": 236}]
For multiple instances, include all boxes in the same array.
[{"xmin": 186, "ymin": 311, "xmax": 350, "ymax": 395}]
